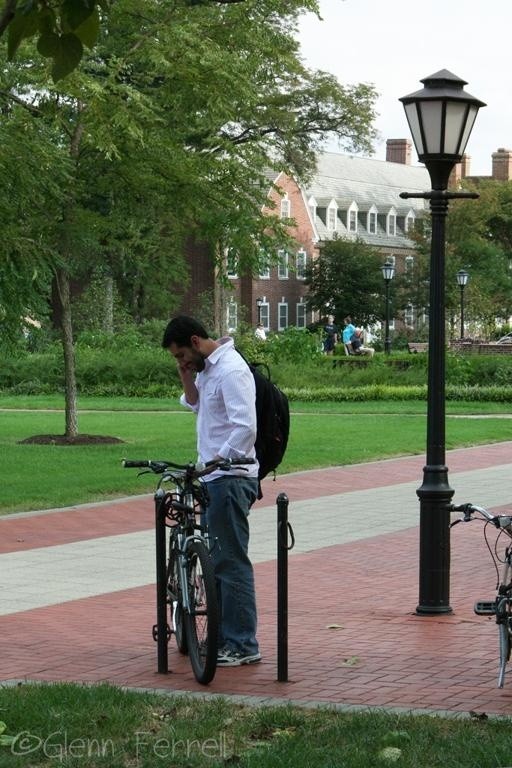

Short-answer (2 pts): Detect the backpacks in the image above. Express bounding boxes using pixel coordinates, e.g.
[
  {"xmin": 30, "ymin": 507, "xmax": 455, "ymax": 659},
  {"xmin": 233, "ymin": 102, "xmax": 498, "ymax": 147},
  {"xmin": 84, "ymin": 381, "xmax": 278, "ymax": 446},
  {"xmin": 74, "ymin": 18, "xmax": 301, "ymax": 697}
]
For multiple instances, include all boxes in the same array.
[{"xmin": 237, "ymin": 348, "xmax": 291, "ymax": 489}]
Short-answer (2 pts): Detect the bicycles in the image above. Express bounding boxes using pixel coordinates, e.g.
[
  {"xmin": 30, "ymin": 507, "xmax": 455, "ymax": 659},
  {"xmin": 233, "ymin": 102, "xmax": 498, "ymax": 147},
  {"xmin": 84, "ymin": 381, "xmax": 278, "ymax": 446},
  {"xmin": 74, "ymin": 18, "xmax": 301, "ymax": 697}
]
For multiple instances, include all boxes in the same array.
[
  {"xmin": 121, "ymin": 457, "xmax": 258, "ymax": 687},
  {"xmin": 441, "ymin": 502, "xmax": 511, "ymax": 686}
]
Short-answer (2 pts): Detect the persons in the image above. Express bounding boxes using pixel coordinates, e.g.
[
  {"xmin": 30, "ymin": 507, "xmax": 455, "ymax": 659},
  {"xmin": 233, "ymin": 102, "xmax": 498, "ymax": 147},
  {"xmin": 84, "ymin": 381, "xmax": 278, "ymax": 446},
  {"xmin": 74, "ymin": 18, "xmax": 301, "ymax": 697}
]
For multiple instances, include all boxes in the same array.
[
  {"xmin": 321, "ymin": 313, "xmax": 374, "ymax": 357},
  {"xmin": 161, "ymin": 317, "xmax": 262, "ymax": 667},
  {"xmin": 255, "ymin": 322, "xmax": 266, "ymax": 342}
]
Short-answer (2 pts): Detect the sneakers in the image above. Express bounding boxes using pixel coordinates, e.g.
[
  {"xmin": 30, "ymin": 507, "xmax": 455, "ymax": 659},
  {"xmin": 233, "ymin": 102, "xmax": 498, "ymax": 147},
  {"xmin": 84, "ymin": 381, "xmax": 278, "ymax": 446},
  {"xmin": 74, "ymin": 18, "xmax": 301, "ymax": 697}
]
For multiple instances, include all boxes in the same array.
[{"xmin": 214, "ymin": 644, "xmax": 264, "ymax": 669}]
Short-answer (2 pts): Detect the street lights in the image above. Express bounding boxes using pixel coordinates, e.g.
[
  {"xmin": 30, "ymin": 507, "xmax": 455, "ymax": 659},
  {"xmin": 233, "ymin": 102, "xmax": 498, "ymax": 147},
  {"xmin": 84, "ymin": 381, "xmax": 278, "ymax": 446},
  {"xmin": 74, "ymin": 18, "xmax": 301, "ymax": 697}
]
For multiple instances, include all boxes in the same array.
[
  {"xmin": 394, "ymin": 70, "xmax": 486, "ymax": 614},
  {"xmin": 451, "ymin": 266, "xmax": 474, "ymax": 341},
  {"xmin": 378, "ymin": 258, "xmax": 399, "ymax": 352}
]
